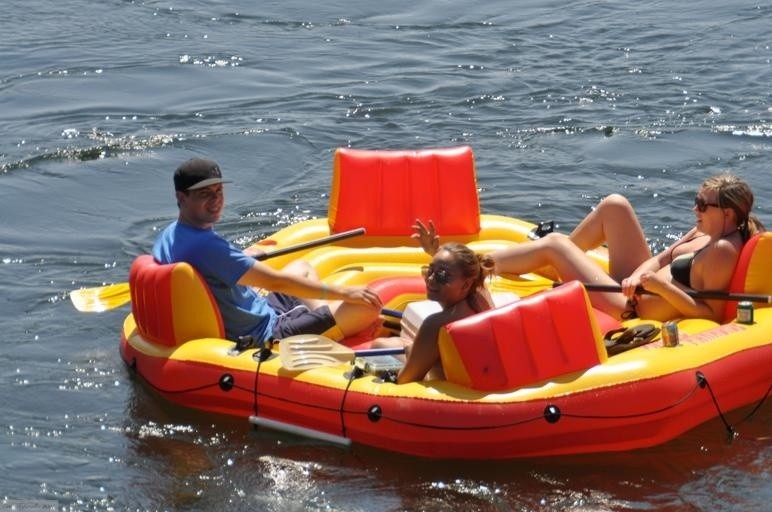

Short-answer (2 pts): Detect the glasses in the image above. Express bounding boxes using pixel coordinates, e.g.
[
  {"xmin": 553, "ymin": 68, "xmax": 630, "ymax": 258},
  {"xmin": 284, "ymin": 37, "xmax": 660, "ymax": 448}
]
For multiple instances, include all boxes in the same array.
[
  {"xmin": 421, "ymin": 265, "xmax": 452, "ymax": 284},
  {"xmin": 694, "ymin": 196, "xmax": 719, "ymax": 212}
]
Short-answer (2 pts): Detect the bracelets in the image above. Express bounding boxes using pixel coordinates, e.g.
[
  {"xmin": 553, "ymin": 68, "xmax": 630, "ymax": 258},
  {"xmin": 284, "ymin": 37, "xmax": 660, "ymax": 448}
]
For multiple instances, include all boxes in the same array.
[{"xmin": 319, "ymin": 281, "xmax": 329, "ymax": 299}]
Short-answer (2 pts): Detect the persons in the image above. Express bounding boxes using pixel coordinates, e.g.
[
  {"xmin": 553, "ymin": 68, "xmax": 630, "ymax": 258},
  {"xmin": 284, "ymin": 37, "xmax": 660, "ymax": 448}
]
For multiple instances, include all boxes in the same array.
[
  {"xmin": 398, "ymin": 218, "xmax": 499, "ymax": 385},
  {"xmin": 152, "ymin": 157, "xmax": 384, "ymax": 347},
  {"xmin": 491, "ymin": 175, "xmax": 765, "ymax": 324}
]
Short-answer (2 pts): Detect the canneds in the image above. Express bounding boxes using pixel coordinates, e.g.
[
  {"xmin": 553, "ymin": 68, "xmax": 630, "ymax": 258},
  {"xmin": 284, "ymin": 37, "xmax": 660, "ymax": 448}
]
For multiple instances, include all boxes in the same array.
[
  {"xmin": 736, "ymin": 301, "xmax": 754, "ymax": 324},
  {"xmin": 661, "ymin": 321, "xmax": 679, "ymax": 347}
]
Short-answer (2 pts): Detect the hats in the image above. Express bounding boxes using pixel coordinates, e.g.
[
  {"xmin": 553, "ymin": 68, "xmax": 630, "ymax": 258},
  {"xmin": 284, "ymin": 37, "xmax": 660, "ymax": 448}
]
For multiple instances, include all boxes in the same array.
[{"xmin": 173, "ymin": 159, "xmax": 233, "ymax": 191}]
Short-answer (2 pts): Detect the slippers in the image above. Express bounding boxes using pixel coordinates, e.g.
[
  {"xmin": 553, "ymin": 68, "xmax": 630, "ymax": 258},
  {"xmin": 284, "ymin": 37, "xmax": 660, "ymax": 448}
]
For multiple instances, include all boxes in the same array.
[{"xmin": 603, "ymin": 324, "xmax": 661, "ymax": 357}]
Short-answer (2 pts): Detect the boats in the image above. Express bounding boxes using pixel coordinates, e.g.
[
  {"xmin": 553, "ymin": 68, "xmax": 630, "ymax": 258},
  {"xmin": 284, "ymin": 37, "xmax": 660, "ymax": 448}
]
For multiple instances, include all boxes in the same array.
[
  {"xmin": 121, "ymin": 146, "xmax": 772, "ymax": 460},
  {"xmin": 123, "ymin": 383, "xmax": 771, "ymax": 511}
]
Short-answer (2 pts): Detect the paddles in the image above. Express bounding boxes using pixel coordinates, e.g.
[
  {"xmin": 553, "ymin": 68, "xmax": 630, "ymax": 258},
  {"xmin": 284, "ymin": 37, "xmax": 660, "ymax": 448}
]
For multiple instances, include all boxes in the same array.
[
  {"xmin": 482, "ymin": 277, "xmax": 770, "ymax": 304},
  {"xmin": 69, "ymin": 227, "xmax": 365, "ymax": 313},
  {"xmin": 281, "ymin": 334, "xmax": 407, "ymax": 370}
]
